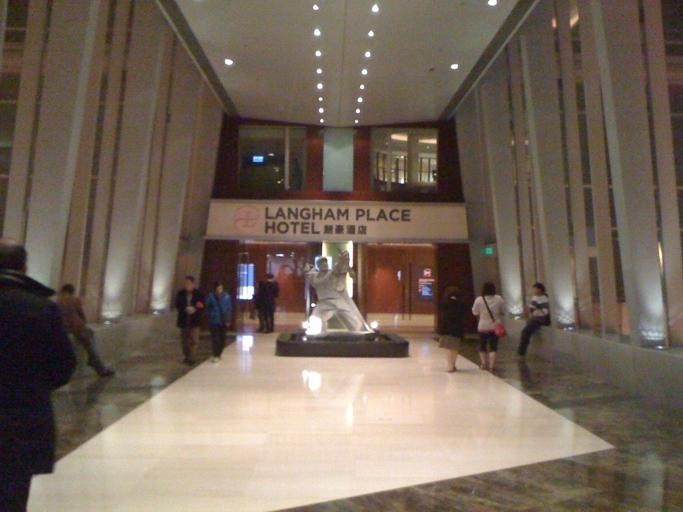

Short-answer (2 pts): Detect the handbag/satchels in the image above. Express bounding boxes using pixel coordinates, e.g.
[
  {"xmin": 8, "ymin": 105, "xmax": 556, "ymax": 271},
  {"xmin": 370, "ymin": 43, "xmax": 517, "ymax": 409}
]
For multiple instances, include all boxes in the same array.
[
  {"xmin": 494, "ymin": 320, "xmax": 509, "ymax": 338},
  {"xmin": 220, "ymin": 316, "xmax": 228, "ymax": 333}
]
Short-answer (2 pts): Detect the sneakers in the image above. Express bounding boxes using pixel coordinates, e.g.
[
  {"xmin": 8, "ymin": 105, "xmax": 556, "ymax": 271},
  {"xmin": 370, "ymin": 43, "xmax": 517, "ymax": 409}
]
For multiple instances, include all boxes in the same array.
[
  {"xmin": 179, "ymin": 351, "xmax": 227, "ymax": 370},
  {"xmin": 86, "ymin": 360, "xmax": 116, "ymax": 379},
  {"xmin": 253, "ymin": 325, "xmax": 275, "ymax": 335}
]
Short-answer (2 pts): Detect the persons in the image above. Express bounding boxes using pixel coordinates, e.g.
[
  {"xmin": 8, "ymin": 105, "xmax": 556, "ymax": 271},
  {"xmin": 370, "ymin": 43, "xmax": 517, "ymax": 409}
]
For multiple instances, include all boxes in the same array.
[
  {"xmin": 0, "ymin": 235, "xmax": 78, "ymax": 511},
  {"xmin": 437, "ymin": 286, "xmax": 465, "ymax": 373},
  {"xmin": 516, "ymin": 282, "xmax": 550, "ymax": 360},
  {"xmin": 471, "ymin": 281, "xmax": 506, "ymax": 373},
  {"xmin": 56, "ymin": 282, "xmax": 114, "ymax": 379},
  {"xmin": 303, "ymin": 248, "xmax": 366, "ymax": 333},
  {"xmin": 197, "ymin": 282, "xmax": 233, "ymax": 362},
  {"xmin": 253, "ymin": 272, "xmax": 281, "ymax": 333},
  {"xmin": 176, "ymin": 276, "xmax": 204, "ymax": 366}
]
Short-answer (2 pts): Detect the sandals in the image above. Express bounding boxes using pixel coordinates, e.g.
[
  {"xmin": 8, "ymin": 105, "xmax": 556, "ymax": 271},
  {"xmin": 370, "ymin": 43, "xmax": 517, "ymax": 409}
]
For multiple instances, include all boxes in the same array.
[{"xmin": 445, "ymin": 353, "xmax": 527, "ymax": 375}]
